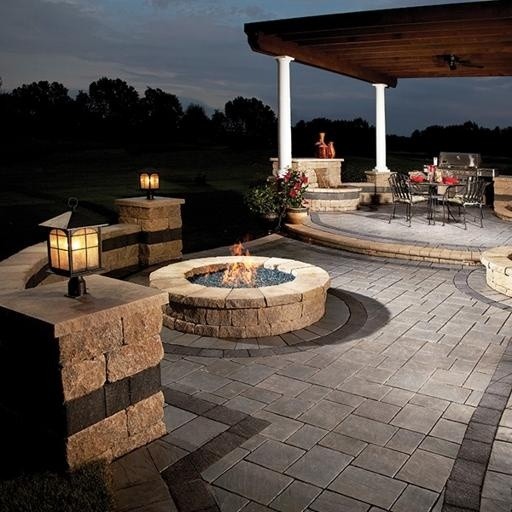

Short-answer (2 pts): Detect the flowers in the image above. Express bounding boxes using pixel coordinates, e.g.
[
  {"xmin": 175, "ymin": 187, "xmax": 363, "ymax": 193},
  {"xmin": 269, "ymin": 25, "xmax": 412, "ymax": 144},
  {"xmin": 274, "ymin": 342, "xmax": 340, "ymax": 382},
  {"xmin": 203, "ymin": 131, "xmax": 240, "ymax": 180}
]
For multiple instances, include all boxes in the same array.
[{"xmin": 278, "ymin": 168, "xmax": 309, "ymax": 208}]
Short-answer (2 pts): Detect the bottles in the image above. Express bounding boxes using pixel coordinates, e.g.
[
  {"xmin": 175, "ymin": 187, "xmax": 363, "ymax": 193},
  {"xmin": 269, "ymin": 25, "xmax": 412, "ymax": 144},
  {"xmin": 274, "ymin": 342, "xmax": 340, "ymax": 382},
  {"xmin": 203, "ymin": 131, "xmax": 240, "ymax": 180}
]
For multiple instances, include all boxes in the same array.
[{"xmin": 427, "ymin": 164, "xmax": 444, "ymax": 183}]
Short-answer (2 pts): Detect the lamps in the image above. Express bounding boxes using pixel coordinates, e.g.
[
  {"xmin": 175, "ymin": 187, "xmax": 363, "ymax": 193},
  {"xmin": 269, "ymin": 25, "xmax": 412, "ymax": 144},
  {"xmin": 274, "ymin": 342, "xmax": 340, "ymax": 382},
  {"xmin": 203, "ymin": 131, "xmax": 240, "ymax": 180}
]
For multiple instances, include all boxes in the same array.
[
  {"xmin": 135, "ymin": 165, "xmax": 162, "ymax": 200},
  {"xmin": 38, "ymin": 196, "xmax": 112, "ymax": 299}
]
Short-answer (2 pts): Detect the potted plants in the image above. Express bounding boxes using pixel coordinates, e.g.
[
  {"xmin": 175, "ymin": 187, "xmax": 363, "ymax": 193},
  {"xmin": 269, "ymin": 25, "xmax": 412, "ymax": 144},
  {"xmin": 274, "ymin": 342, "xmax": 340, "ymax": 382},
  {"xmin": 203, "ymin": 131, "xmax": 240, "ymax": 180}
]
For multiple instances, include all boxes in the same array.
[{"xmin": 244, "ymin": 176, "xmax": 279, "ymax": 230}]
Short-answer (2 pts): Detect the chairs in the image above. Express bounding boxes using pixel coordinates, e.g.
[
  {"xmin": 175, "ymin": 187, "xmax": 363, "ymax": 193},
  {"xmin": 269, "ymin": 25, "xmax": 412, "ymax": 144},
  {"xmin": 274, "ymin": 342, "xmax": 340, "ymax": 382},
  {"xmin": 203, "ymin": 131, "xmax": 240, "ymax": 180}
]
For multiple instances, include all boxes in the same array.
[{"xmin": 388, "ymin": 164, "xmax": 493, "ymax": 230}]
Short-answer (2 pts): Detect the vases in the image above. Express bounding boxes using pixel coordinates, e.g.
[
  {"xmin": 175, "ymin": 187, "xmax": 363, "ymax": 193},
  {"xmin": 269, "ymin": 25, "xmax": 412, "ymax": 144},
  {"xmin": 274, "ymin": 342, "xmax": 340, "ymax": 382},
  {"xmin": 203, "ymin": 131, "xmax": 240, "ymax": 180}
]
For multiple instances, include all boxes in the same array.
[{"xmin": 285, "ymin": 206, "xmax": 308, "ymax": 224}]
